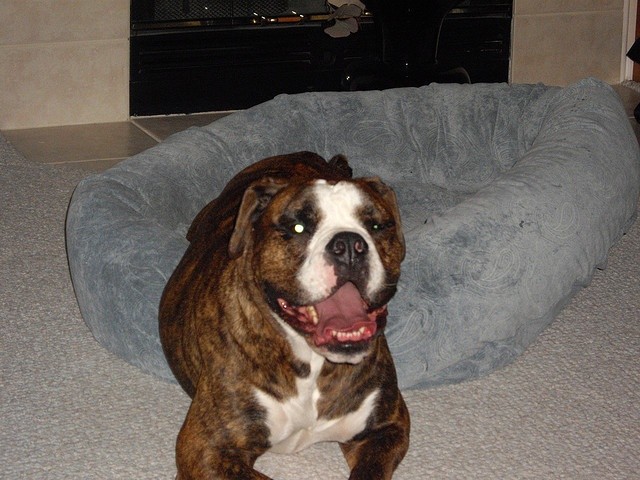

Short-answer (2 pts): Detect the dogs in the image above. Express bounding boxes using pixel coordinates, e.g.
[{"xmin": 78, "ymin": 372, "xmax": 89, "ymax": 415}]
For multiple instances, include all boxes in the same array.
[{"xmin": 157, "ymin": 149, "xmax": 412, "ymax": 480}]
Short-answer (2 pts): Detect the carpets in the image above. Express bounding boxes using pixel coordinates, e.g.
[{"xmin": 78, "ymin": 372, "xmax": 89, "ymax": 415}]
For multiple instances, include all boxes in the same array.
[{"xmin": 2, "ymin": 130, "xmax": 640, "ymax": 480}]
